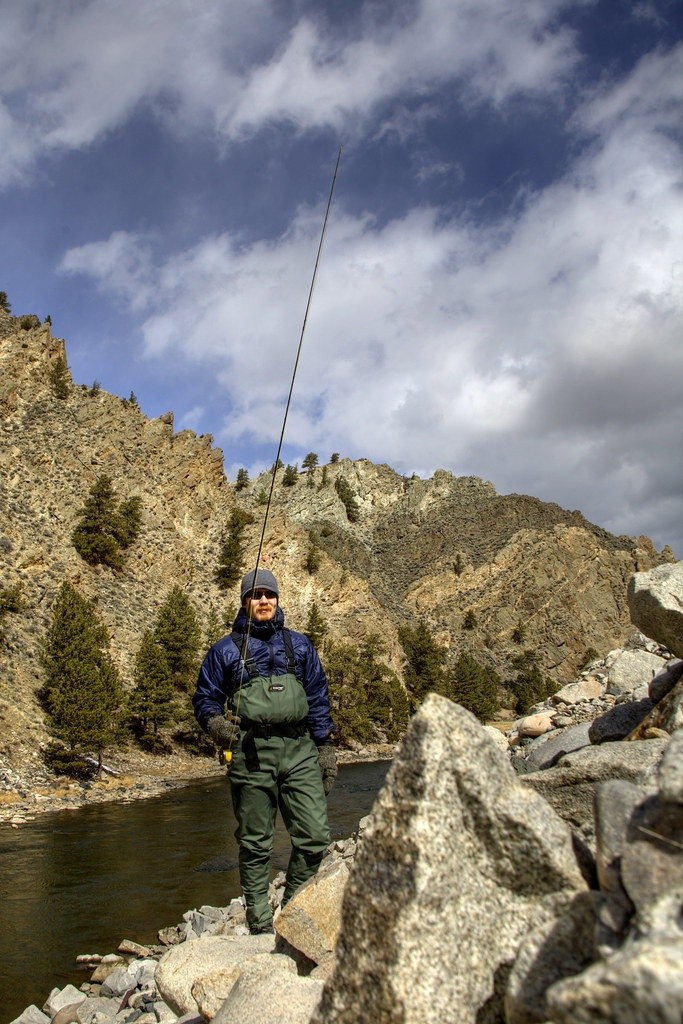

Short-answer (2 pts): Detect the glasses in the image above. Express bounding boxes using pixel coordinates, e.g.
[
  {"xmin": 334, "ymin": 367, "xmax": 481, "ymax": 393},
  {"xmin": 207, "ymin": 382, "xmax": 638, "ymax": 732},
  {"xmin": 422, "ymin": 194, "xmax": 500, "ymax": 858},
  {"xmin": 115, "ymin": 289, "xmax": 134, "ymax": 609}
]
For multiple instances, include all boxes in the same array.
[{"xmin": 246, "ymin": 590, "xmax": 276, "ymax": 599}]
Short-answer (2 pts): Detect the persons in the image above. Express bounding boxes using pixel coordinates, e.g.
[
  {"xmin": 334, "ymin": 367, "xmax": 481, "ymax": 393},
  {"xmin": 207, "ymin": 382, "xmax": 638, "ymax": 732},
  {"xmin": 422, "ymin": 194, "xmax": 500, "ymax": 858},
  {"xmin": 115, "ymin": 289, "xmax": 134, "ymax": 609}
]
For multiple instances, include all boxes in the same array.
[{"xmin": 194, "ymin": 570, "xmax": 338, "ymax": 935}]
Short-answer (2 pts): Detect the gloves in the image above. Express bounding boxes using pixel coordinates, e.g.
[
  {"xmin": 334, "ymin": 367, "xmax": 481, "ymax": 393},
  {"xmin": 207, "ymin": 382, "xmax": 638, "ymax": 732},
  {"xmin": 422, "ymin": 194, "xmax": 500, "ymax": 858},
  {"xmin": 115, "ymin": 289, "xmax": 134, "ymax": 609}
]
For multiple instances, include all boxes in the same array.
[
  {"xmin": 207, "ymin": 714, "xmax": 241, "ymax": 747},
  {"xmin": 317, "ymin": 746, "xmax": 338, "ymax": 795}
]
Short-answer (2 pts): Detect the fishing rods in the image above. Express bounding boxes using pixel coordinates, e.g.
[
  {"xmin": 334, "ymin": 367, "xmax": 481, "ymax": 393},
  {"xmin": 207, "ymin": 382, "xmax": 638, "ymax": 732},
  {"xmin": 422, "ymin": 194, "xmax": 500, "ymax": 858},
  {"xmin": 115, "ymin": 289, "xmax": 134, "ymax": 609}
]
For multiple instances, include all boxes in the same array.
[{"xmin": 218, "ymin": 143, "xmax": 346, "ymax": 767}]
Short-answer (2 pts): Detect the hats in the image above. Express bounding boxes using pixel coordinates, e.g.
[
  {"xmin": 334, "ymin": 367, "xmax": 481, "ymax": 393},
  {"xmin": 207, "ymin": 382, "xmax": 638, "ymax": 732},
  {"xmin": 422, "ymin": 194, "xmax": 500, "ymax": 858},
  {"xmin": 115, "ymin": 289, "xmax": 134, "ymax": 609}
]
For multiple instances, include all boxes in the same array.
[{"xmin": 241, "ymin": 569, "xmax": 279, "ymax": 600}]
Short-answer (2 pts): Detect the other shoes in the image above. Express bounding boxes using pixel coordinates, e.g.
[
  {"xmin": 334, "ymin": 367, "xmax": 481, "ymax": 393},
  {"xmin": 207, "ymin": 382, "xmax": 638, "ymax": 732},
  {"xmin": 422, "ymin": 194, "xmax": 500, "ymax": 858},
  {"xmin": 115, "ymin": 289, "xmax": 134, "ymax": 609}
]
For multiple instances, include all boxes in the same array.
[{"xmin": 250, "ymin": 921, "xmax": 275, "ymax": 935}]
